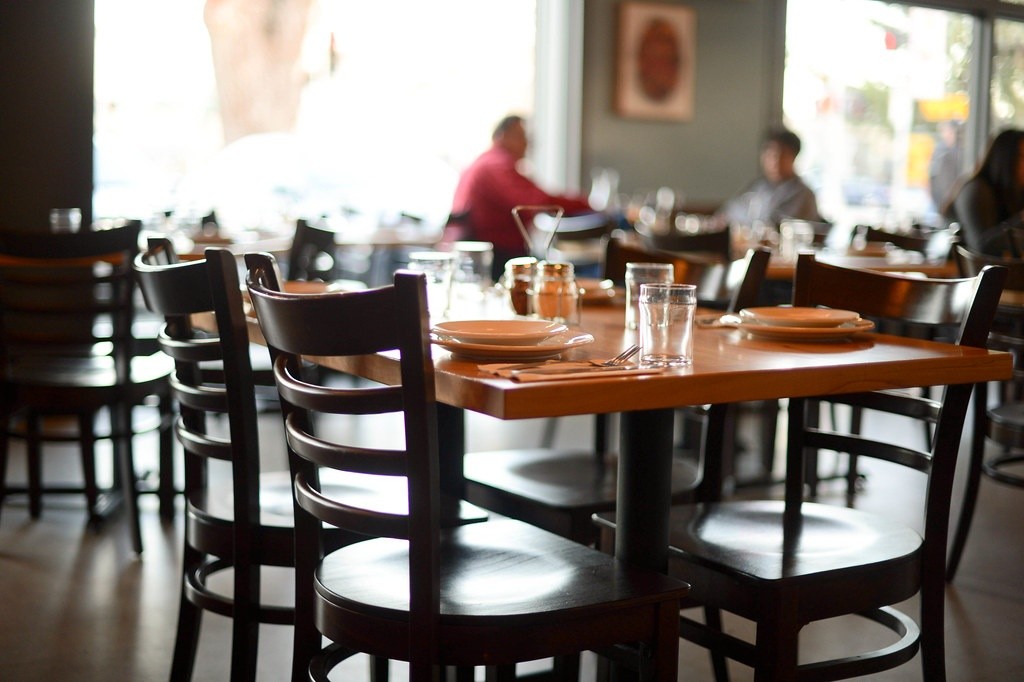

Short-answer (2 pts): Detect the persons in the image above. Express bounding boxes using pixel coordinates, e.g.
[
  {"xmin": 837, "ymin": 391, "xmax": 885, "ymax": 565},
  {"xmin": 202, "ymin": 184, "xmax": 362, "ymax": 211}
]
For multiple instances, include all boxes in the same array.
[
  {"xmin": 943, "ymin": 129, "xmax": 1024, "ymax": 285},
  {"xmin": 710, "ymin": 130, "xmax": 819, "ymax": 309},
  {"xmin": 434, "ymin": 116, "xmax": 592, "ymax": 282}
]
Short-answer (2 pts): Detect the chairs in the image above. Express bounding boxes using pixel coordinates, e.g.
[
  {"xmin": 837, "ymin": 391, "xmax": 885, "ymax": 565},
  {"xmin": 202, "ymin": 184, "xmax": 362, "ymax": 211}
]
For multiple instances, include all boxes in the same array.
[{"xmin": 0, "ymin": 201, "xmax": 1024, "ymax": 682}]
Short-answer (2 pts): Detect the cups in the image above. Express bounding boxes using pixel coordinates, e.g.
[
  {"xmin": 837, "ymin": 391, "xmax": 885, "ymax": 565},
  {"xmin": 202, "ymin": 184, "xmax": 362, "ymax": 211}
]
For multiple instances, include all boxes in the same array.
[
  {"xmin": 404, "ymin": 239, "xmax": 495, "ymax": 322},
  {"xmin": 638, "ymin": 283, "xmax": 697, "ymax": 368},
  {"xmin": 49, "ymin": 208, "xmax": 81, "ymax": 235},
  {"xmin": 623, "ymin": 262, "xmax": 675, "ymax": 330}
]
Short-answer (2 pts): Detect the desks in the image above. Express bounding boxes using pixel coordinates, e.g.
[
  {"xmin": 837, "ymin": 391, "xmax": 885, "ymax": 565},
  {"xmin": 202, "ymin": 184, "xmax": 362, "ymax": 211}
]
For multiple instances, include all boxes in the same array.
[
  {"xmin": 204, "ymin": 225, "xmax": 440, "ymax": 277},
  {"xmin": 615, "ymin": 236, "xmax": 947, "ymax": 323},
  {"xmin": 182, "ymin": 279, "xmax": 1015, "ymax": 682}
]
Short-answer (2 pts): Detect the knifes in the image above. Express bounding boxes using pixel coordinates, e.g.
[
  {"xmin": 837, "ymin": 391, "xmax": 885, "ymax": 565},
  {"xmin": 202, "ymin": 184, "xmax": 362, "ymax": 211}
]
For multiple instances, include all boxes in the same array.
[{"xmin": 511, "ymin": 365, "xmax": 660, "ymax": 374}]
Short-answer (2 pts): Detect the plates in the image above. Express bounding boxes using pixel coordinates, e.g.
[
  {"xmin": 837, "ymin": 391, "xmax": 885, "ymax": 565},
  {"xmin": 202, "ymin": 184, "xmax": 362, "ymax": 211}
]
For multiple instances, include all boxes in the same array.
[
  {"xmin": 738, "ymin": 306, "xmax": 875, "ymax": 341},
  {"xmin": 502, "ymin": 256, "xmax": 577, "ymax": 322},
  {"xmin": 429, "ymin": 320, "xmax": 598, "ymax": 362},
  {"xmin": 577, "ymin": 277, "xmax": 615, "ymax": 303}
]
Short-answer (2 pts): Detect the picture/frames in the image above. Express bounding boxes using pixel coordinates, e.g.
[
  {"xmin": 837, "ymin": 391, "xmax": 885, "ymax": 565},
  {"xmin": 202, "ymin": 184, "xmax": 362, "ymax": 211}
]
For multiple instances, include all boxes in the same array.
[{"xmin": 610, "ymin": 3, "xmax": 698, "ymax": 123}]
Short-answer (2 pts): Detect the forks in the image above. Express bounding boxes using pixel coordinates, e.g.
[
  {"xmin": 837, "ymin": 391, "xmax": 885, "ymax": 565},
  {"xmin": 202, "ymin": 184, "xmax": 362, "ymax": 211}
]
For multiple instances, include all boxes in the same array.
[{"xmin": 499, "ymin": 344, "xmax": 641, "ymax": 372}]
